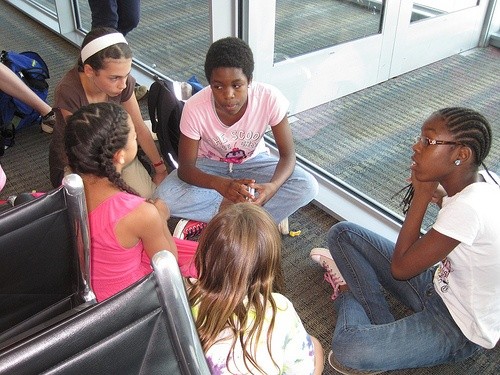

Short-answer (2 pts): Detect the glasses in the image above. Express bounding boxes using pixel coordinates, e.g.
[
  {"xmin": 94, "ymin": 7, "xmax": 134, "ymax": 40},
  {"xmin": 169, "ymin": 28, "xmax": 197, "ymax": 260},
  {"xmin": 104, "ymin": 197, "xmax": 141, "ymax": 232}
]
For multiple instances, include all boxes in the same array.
[{"xmin": 416, "ymin": 135, "xmax": 460, "ymax": 147}]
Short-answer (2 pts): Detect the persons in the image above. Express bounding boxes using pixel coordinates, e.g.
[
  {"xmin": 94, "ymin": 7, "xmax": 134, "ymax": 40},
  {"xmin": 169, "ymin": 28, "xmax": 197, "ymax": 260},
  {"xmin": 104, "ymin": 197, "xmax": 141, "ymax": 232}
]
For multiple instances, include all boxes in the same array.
[
  {"xmin": 311, "ymin": 106, "xmax": 500, "ymax": 375},
  {"xmin": 189, "ymin": 202, "xmax": 326, "ymax": 375},
  {"xmin": 64, "ymin": 101, "xmax": 199, "ymax": 304},
  {"xmin": 49, "ymin": 28, "xmax": 168, "ymax": 201},
  {"xmin": 0, "ymin": 49, "xmax": 57, "ymax": 155},
  {"xmin": 151, "ymin": 37, "xmax": 319, "ymax": 236},
  {"xmin": 88, "ymin": 0, "xmax": 141, "ymax": 38}
]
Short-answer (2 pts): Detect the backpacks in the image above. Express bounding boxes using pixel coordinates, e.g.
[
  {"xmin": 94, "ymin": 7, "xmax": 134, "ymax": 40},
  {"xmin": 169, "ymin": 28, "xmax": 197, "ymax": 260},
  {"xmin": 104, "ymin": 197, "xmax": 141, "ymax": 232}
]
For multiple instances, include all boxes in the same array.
[
  {"xmin": 1, "ymin": 49, "xmax": 50, "ymax": 133},
  {"xmin": 147, "ymin": 74, "xmax": 206, "ymax": 168}
]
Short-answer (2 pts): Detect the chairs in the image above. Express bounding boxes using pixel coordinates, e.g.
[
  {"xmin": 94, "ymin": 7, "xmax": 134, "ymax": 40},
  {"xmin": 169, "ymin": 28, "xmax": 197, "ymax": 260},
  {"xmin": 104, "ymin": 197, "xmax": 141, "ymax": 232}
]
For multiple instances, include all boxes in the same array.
[
  {"xmin": 0, "ymin": 250, "xmax": 211, "ymax": 375},
  {"xmin": 0, "ymin": 173, "xmax": 97, "ymax": 349}
]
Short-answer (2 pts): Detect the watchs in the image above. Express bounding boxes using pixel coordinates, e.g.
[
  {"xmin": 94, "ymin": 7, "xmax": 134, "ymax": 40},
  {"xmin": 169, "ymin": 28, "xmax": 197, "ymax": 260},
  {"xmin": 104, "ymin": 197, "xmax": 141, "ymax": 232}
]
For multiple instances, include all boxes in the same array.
[{"xmin": 150, "ymin": 157, "xmax": 165, "ymax": 168}]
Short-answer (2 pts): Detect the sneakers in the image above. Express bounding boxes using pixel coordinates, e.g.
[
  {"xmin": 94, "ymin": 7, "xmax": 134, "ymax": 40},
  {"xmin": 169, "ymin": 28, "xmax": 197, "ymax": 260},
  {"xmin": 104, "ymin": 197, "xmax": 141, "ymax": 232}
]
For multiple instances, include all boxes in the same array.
[
  {"xmin": 278, "ymin": 215, "xmax": 291, "ymax": 237},
  {"xmin": 41, "ymin": 109, "xmax": 58, "ymax": 135},
  {"xmin": 308, "ymin": 247, "xmax": 350, "ymax": 299},
  {"xmin": 327, "ymin": 350, "xmax": 389, "ymax": 375},
  {"xmin": 173, "ymin": 216, "xmax": 210, "ymax": 241}
]
